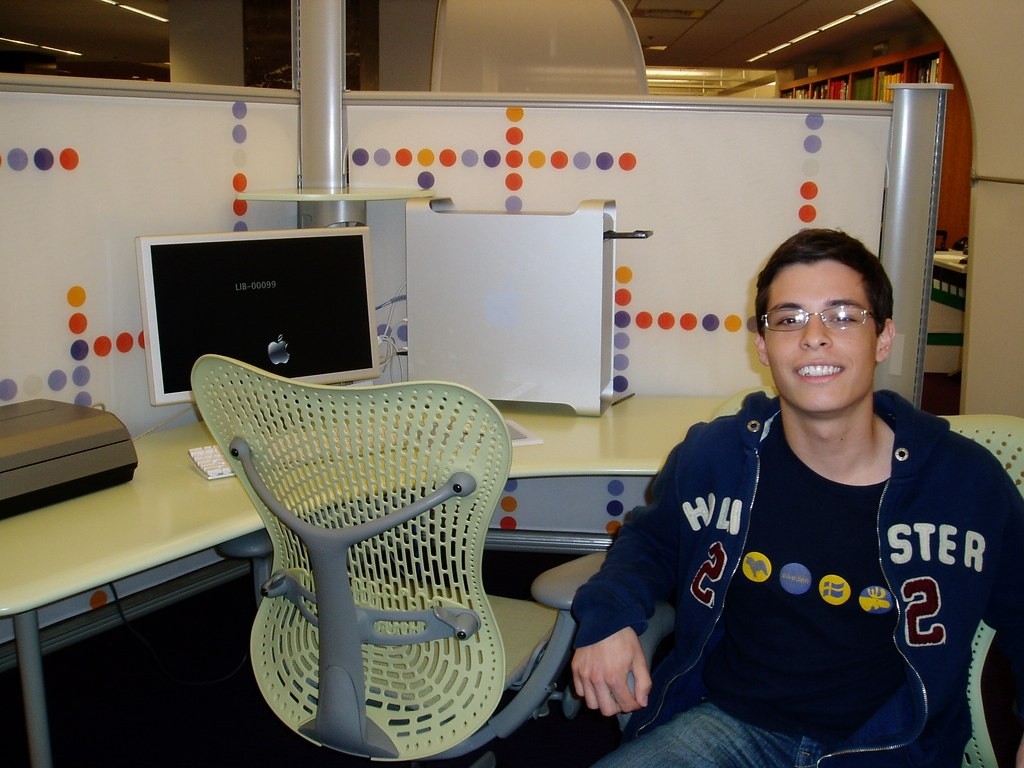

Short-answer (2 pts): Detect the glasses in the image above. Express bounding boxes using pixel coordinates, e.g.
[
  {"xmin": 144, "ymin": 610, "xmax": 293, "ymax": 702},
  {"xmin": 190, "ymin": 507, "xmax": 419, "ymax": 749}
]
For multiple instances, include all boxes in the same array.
[{"xmin": 761, "ymin": 303, "xmax": 883, "ymax": 332}]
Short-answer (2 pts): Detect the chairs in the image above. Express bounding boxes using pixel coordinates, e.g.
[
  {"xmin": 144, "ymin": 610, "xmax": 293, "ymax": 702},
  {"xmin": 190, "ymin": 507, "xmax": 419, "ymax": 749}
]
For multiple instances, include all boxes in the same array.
[
  {"xmin": 621, "ymin": 413, "xmax": 1024, "ymax": 768},
  {"xmin": 190, "ymin": 354, "xmax": 606, "ymax": 768}
]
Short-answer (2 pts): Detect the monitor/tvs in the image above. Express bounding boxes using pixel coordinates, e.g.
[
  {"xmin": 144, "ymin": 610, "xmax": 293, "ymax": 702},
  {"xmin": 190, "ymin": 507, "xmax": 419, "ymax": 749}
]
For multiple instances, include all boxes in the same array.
[{"xmin": 138, "ymin": 225, "xmax": 379, "ymax": 405}]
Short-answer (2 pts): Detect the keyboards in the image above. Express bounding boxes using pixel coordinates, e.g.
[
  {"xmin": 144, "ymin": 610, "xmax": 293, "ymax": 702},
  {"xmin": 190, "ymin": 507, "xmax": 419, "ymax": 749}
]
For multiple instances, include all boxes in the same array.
[{"xmin": 187, "ymin": 423, "xmax": 419, "ymax": 480}]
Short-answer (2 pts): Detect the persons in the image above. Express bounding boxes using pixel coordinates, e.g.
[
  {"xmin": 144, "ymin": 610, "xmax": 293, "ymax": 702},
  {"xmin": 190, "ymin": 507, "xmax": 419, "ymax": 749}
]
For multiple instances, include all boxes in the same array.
[{"xmin": 572, "ymin": 227, "xmax": 1024, "ymax": 768}]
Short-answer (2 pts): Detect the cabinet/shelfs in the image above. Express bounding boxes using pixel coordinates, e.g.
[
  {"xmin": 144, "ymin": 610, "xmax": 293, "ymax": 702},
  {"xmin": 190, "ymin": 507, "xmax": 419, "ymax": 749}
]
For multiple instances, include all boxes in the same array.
[{"xmin": 779, "ymin": 42, "xmax": 971, "ymax": 249}]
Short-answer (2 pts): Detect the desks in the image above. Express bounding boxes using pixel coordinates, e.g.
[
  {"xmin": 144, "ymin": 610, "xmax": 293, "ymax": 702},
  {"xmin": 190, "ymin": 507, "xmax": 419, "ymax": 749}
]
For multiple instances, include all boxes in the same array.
[{"xmin": 0, "ymin": 396, "xmax": 726, "ymax": 768}]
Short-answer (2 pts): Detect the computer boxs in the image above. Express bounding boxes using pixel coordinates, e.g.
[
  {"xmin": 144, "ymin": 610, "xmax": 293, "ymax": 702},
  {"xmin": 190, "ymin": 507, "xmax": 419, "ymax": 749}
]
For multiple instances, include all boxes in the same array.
[{"xmin": 406, "ymin": 197, "xmax": 618, "ymax": 415}]
